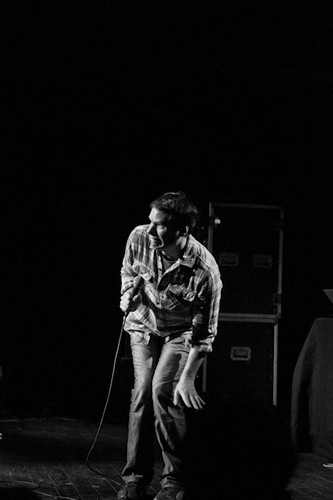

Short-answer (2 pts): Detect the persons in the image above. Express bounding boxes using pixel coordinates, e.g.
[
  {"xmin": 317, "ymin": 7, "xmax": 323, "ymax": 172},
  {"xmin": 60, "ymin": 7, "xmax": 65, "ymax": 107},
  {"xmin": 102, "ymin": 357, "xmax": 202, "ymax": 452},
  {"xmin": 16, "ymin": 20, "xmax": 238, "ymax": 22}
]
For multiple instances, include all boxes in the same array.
[{"xmin": 117, "ymin": 191, "xmax": 223, "ymax": 500}]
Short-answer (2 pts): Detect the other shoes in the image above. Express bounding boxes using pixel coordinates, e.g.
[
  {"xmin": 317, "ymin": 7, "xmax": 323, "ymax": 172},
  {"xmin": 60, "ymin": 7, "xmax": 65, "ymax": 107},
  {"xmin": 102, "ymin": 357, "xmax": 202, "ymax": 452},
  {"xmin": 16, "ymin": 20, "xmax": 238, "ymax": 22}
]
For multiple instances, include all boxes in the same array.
[
  {"xmin": 152, "ymin": 488, "xmax": 182, "ymax": 500},
  {"xmin": 117, "ymin": 481, "xmax": 150, "ymax": 500}
]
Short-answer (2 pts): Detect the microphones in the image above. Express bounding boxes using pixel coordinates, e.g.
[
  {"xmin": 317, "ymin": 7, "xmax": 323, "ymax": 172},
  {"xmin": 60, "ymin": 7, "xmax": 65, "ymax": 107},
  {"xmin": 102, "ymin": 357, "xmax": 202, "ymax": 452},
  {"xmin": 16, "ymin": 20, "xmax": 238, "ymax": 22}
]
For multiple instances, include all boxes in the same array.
[{"xmin": 126, "ymin": 276, "xmax": 146, "ymax": 314}]
